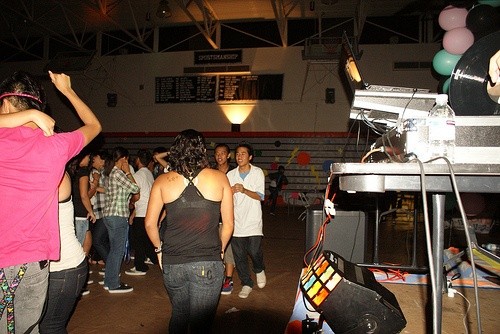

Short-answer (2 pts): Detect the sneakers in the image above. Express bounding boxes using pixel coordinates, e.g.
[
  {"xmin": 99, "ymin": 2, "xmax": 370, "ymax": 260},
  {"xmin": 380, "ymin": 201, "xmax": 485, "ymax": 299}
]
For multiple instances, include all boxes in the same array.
[
  {"xmin": 256, "ymin": 270, "xmax": 266, "ymax": 289},
  {"xmin": 219, "ymin": 276, "xmax": 234, "ymax": 295},
  {"xmin": 238, "ymin": 284, "xmax": 252, "ymax": 298}
]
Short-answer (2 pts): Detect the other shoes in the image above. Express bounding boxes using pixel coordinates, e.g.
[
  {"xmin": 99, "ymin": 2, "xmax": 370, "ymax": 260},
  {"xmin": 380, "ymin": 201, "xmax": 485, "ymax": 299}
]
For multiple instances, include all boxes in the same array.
[
  {"xmin": 109, "ymin": 284, "xmax": 134, "ymax": 294},
  {"xmin": 144, "ymin": 258, "xmax": 154, "ymax": 265},
  {"xmin": 81, "ymin": 290, "xmax": 90, "ymax": 295},
  {"xmin": 98, "ymin": 260, "xmax": 105, "ymax": 266},
  {"xmin": 103, "ymin": 282, "xmax": 127, "ymax": 290},
  {"xmin": 98, "ymin": 269, "xmax": 105, "ymax": 275},
  {"xmin": 125, "ymin": 267, "xmax": 147, "ymax": 276},
  {"xmin": 89, "ymin": 258, "xmax": 98, "ymax": 264}
]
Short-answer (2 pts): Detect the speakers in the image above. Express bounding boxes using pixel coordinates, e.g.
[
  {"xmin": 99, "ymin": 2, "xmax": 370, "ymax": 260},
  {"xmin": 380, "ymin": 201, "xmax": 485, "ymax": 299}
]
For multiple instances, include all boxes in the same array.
[{"xmin": 300, "ymin": 250, "xmax": 407, "ymax": 334}]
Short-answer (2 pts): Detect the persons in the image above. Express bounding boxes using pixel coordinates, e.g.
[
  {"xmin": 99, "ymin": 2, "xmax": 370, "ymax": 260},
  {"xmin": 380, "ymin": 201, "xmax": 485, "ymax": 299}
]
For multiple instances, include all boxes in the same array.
[
  {"xmin": 380, "ymin": 191, "xmax": 398, "ymax": 224},
  {"xmin": 67, "ymin": 144, "xmax": 176, "ymax": 295},
  {"xmin": 211, "ymin": 143, "xmax": 237, "ymax": 295},
  {"xmin": 0, "ymin": 109, "xmax": 89, "ymax": 334},
  {"xmin": 265, "ymin": 165, "xmax": 288, "ymax": 214},
  {"xmin": 145, "ymin": 129, "xmax": 233, "ymax": 334},
  {"xmin": 227, "ymin": 144, "xmax": 266, "ymax": 298},
  {"xmin": 0, "ymin": 71, "xmax": 102, "ymax": 334}
]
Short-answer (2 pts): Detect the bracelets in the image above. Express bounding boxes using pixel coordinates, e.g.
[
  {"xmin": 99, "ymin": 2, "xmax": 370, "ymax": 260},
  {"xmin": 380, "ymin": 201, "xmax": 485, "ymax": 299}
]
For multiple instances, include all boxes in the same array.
[
  {"xmin": 94, "ymin": 179, "xmax": 98, "ymax": 182},
  {"xmin": 167, "ymin": 152, "xmax": 168, "ymax": 155},
  {"xmin": 155, "ymin": 250, "xmax": 162, "ymax": 253},
  {"xmin": 155, "ymin": 247, "xmax": 160, "ymax": 249},
  {"xmin": 126, "ymin": 172, "xmax": 131, "ymax": 176}
]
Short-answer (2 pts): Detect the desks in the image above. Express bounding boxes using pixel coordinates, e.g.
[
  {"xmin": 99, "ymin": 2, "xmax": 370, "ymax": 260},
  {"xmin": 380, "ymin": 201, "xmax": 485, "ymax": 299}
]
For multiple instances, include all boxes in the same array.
[{"xmin": 330, "ymin": 163, "xmax": 500, "ymax": 334}]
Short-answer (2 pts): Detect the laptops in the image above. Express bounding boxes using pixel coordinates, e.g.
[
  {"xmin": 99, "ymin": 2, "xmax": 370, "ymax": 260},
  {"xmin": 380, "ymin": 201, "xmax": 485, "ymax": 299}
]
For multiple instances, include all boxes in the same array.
[{"xmin": 339, "ymin": 30, "xmax": 430, "ymax": 95}]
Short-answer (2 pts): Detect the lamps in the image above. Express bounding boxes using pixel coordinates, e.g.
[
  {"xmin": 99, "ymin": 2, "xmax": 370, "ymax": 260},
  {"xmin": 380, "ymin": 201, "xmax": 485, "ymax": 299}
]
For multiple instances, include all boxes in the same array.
[{"xmin": 156, "ymin": 0, "xmax": 172, "ymax": 18}]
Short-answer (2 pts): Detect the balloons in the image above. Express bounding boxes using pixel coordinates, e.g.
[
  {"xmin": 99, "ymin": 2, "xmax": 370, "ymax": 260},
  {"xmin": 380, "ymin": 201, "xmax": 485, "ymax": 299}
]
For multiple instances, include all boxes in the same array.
[
  {"xmin": 466, "ymin": 4, "xmax": 499, "ymax": 32},
  {"xmin": 443, "ymin": 78, "xmax": 449, "ymax": 94},
  {"xmin": 439, "ymin": 5, "xmax": 468, "ymax": 31},
  {"xmin": 478, "ymin": 0, "xmax": 500, "ymax": 7},
  {"xmin": 433, "ymin": 50, "xmax": 464, "ymax": 76},
  {"xmin": 443, "ymin": 27, "xmax": 474, "ymax": 54}
]
